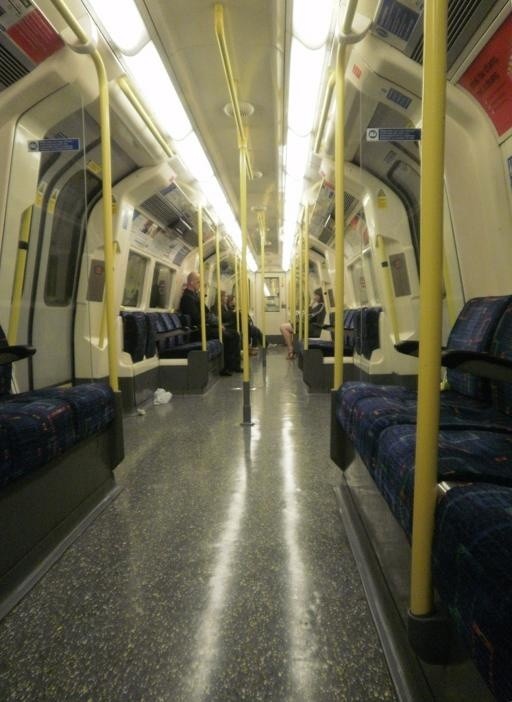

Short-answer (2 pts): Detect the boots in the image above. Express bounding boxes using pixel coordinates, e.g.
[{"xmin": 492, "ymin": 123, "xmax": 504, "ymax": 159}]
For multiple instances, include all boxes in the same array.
[{"xmin": 249, "ymin": 344, "xmax": 257, "ymax": 356}]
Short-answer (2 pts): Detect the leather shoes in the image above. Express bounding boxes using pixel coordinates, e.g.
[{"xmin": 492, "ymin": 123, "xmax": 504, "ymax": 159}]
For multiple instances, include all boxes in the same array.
[{"xmin": 218, "ymin": 366, "xmax": 243, "ymax": 377}]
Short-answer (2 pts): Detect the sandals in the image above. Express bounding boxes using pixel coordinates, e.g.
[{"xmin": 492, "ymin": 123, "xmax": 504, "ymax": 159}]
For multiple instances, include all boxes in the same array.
[{"xmin": 288, "ymin": 351, "xmax": 296, "ymax": 359}]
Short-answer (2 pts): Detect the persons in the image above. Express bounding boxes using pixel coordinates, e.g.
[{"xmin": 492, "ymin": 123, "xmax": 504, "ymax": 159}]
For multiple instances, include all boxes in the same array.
[
  {"xmin": 150, "ymin": 266, "xmax": 160, "ymax": 307},
  {"xmin": 210, "ymin": 289, "xmax": 252, "ymax": 353},
  {"xmin": 179, "ymin": 271, "xmax": 244, "ymax": 376},
  {"xmin": 279, "ymin": 287, "xmax": 327, "ymax": 360},
  {"xmin": 158, "ymin": 279, "xmax": 168, "ymax": 307},
  {"xmin": 225, "ymin": 293, "xmax": 278, "ymax": 353},
  {"xmin": 181, "ymin": 283, "xmax": 188, "ymax": 295}
]
